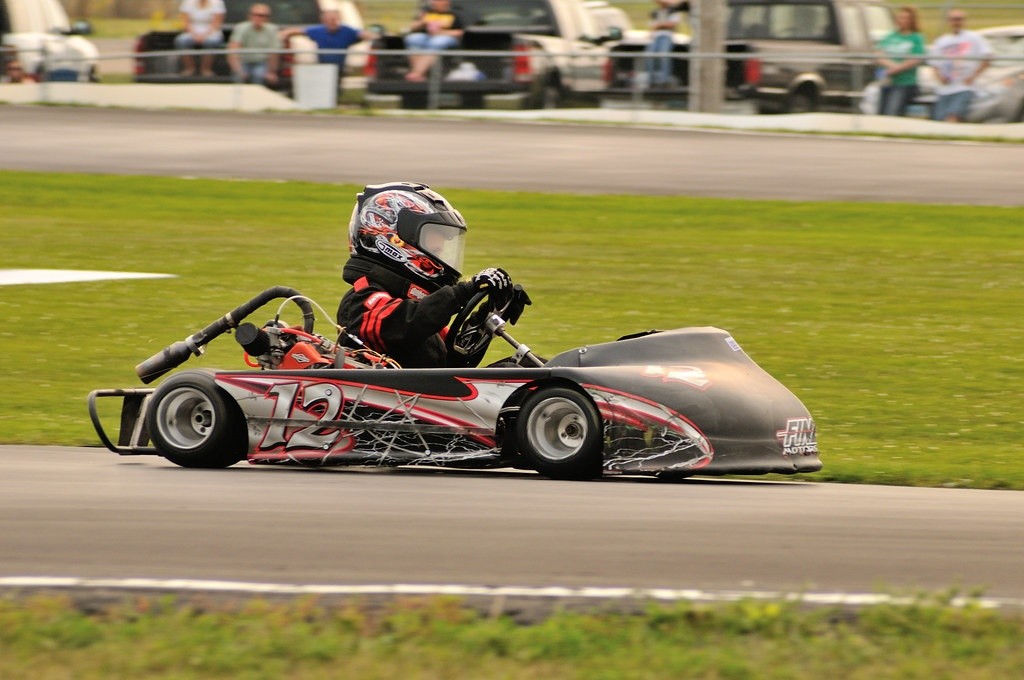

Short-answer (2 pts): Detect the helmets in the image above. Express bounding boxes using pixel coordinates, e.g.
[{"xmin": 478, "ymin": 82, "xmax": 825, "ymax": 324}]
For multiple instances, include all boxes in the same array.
[{"xmin": 342, "ymin": 181, "xmax": 468, "ymax": 289}]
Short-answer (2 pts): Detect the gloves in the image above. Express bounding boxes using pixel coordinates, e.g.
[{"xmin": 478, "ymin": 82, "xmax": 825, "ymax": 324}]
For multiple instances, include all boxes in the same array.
[{"xmin": 457, "ymin": 268, "xmax": 513, "ymax": 311}]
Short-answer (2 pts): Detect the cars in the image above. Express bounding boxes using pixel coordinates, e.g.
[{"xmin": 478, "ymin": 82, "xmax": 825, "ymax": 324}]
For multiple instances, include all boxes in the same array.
[
  {"xmin": 0, "ymin": 0, "xmax": 102, "ymax": 84},
  {"xmin": 863, "ymin": 20, "xmax": 1024, "ymax": 126}
]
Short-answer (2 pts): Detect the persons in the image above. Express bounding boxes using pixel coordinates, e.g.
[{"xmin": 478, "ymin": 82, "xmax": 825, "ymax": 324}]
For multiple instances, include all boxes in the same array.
[
  {"xmin": 226, "ymin": 4, "xmax": 281, "ymax": 87},
  {"xmin": 928, "ymin": 10, "xmax": 994, "ymax": 123},
  {"xmin": 404, "ymin": 0, "xmax": 465, "ymax": 82},
  {"xmin": 873, "ymin": 7, "xmax": 928, "ymax": 116},
  {"xmin": 174, "ymin": 0, "xmax": 227, "ymax": 78},
  {"xmin": 654, "ymin": 1, "xmax": 693, "ymax": 34},
  {"xmin": 337, "ymin": 182, "xmax": 548, "ymax": 368},
  {"xmin": 282, "ymin": 10, "xmax": 397, "ymax": 83}
]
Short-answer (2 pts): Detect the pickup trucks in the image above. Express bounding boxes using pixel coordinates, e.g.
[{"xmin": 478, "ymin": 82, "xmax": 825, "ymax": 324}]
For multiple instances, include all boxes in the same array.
[
  {"xmin": 363, "ymin": 0, "xmax": 634, "ymax": 110},
  {"xmin": 606, "ymin": 2, "xmax": 906, "ymax": 113},
  {"xmin": 136, "ymin": 2, "xmax": 382, "ymax": 87}
]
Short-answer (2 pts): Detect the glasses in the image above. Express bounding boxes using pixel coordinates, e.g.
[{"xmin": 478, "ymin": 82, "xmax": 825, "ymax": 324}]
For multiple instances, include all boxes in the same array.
[{"xmin": 252, "ymin": 13, "xmax": 271, "ymax": 18}]
